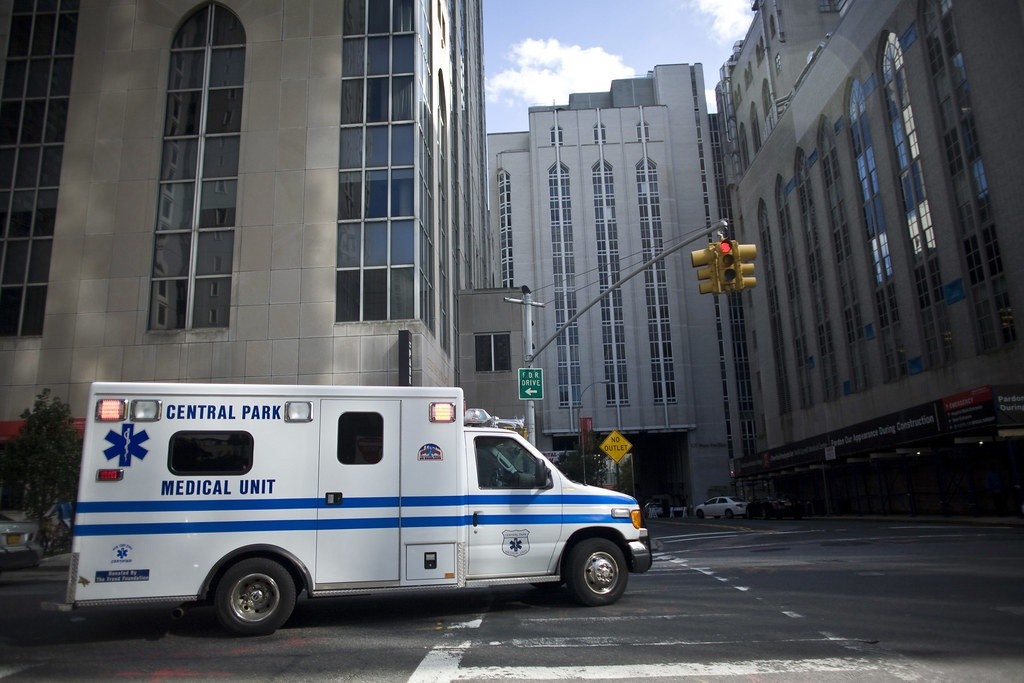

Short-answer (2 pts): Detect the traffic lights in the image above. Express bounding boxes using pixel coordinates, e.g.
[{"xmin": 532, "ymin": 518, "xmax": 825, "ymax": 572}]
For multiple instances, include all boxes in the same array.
[
  {"xmin": 736, "ymin": 243, "xmax": 757, "ymax": 289},
  {"xmin": 717, "ymin": 239, "xmax": 741, "ymax": 290},
  {"xmin": 691, "ymin": 244, "xmax": 720, "ymax": 295}
]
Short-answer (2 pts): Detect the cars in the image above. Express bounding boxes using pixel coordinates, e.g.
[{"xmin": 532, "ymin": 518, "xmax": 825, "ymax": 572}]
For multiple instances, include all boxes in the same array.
[
  {"xmin": 748, "ymin": 497, "xmax": 802, "ymax": 521},
  {"xmin": 0, "ymin": 513, "xmax": 44, "ymax": 570},
  {"xmin": 694, "ymin": 496, "xmax": 749, "ymax": 518},
  {"xmin": 643, "ymin": 503, "xmax": 665, "ymax": 520}
]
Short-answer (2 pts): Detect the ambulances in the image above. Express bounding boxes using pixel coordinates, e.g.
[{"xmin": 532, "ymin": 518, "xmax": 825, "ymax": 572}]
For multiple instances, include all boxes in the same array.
[{"xmin": 67, "ymin": 381, "xmax": 652, "ymax": 632}]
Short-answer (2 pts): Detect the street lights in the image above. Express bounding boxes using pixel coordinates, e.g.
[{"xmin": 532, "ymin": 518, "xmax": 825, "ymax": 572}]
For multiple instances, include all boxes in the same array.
[{"xmin": 578, "ymin": 380, "xmax": 609, "ymax": 485}]
